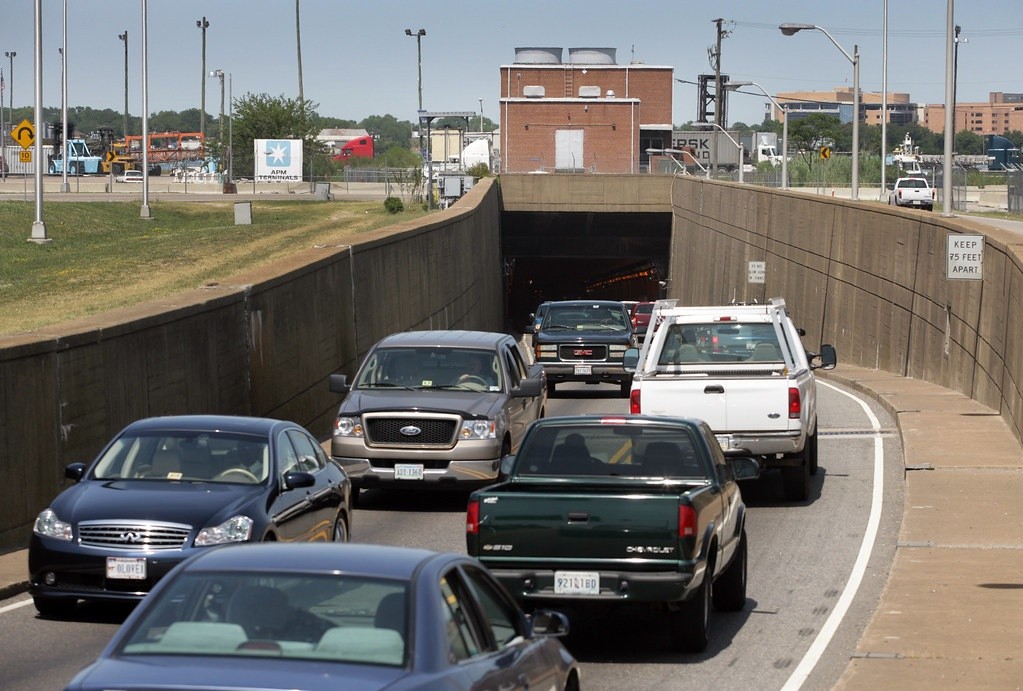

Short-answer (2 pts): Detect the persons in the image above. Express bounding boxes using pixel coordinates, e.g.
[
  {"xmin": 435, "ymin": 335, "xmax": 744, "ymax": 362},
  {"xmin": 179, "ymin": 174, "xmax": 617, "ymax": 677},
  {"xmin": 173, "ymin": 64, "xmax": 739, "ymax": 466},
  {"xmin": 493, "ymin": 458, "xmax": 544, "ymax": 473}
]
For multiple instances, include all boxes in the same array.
[
  {"xmin": 224, "ymin": 441, "xmax": 263, "ymax": 481},
  {"xmin": 458, "ymin": 357, "xmax": 494, "ymax": 387},
  {"xmin": 680, "ymin": 330, "xmax": 712, "ymax": 361},
  {"xmin": 564, "ymin": 432, "xmax": 598, "ymax": 464}
]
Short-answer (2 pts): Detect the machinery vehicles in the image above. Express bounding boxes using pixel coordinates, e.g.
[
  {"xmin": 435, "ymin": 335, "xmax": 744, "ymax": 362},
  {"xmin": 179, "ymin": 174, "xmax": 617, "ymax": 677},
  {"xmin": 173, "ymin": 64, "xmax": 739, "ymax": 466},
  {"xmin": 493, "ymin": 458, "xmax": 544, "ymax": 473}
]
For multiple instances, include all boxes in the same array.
[{"xmin": 45, "ymin": 121, "xmax": 136, "ymax": 176}]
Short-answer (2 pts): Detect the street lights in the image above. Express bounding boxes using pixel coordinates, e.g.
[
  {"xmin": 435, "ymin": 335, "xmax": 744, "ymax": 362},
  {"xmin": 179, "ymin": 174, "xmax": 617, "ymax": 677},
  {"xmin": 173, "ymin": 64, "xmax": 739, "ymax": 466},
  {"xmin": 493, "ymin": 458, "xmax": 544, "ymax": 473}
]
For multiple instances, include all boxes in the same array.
[
  {"xmin": 645, "ymin": 120, "xmax": 744, "ymax": 184},
  {"xmin": 5, "ymin": 51, "xmax": 17, "ymax": 122},
  {"xmin": 725, "ymin": 80, "xmax": 788, "ymax": 189},
  {"xmin": 196, "ymin": 16, "xmax": 210, "ymax": 158},
  {"xmin": 207, "ymin": 69, "xmax": 225, "ymax": 142},
  {"xmin": 406, "ymin": 29, "xmax": 426, "ymax": 110},
  {"xmin": 118, "ymin": 31, "xmax": 128, "ymax": 136},
  {"xmin": 779, "ymin": 23, "xmax": 860, "ymax": 200}
]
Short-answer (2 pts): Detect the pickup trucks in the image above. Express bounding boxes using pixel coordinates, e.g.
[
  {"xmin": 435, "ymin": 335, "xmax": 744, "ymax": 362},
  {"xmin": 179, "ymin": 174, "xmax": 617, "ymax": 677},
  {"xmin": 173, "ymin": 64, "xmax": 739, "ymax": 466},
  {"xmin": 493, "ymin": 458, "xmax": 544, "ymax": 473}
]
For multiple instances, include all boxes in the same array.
[
  {"xmin": 630, "ymin": 297, "xmax": 837, "ymax": 504},
  {"xmin": 328, "ymin": 330, "xmax": 548, "ymax": 502},
  {"xmin": 888, "ymin": 177, "xmax": 935, "ymax": 213},
  {"xmin": 465, "ymin": 413, "xmax": 762, "ymax": 653}
]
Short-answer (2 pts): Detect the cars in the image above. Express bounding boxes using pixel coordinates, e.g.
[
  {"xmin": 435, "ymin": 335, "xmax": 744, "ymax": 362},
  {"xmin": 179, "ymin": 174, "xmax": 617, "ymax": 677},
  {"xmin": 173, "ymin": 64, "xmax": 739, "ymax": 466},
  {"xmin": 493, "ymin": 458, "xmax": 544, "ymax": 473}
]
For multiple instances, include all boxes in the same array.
[
  {"xmin": 533, "ymin": 300, "xmax": 638, "ymax": 398},
  {"xmin": 528, "ymin": 298, "xmax": 683, "ymax": 343},
  {"xmin": 62, "ymin": 539, "xmax": 581, "ymax": 691},
  {"xmin": 28, "ymin": 415, "xmax": 353, "ymax": 620}
]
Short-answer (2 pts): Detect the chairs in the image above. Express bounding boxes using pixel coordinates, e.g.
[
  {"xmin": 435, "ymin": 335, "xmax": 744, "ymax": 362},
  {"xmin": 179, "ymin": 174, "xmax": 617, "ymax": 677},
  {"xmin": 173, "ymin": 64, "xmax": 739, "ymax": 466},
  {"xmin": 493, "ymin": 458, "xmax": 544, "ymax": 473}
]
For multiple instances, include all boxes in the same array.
[
  {"xmin": 149, "ymin": 450, "xmax": 182, "ymax": 477},
  {"xmin": 177, "ymin": 439, "xmax": 213, "ymax": 478},
  {"xmin": 744, "ymin": 342, "xmax": 782, "ymax": 361},
  {"xmin": 670, "ymin": 344, "xmax": 702, "ymax": 362},
  {"xmin": 158, "ymin": 585, "xmax": 405, "ymax": 666},
  {"xmin": 385, "ymin": 354, "xmax": 423, "ymax": 384},
  {"xmin": 641, "ymin": 441, "xmax": 687, "ymax": 475},
  {"xmin": 543, "ymin": 444, "xmax": 589, "ymax": 475}
]
